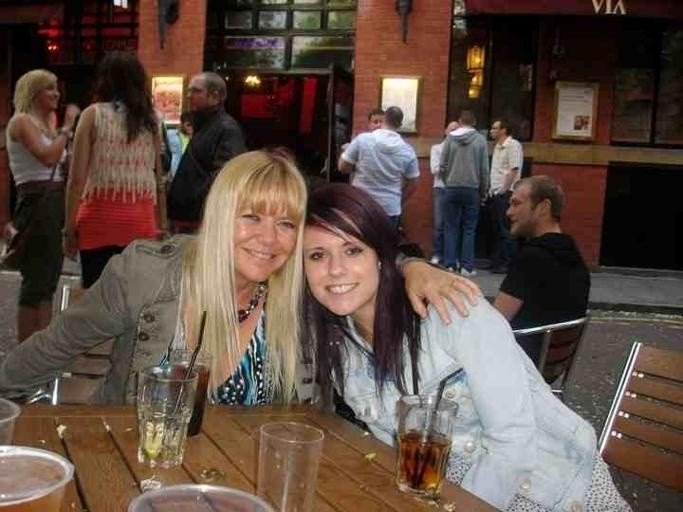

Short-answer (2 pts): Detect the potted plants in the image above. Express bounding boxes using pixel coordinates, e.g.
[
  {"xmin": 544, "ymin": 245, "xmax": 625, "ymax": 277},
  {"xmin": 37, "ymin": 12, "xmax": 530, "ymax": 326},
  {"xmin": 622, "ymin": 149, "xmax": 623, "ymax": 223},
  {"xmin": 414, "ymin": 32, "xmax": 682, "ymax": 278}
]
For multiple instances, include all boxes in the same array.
[{"xmin": 551, "ymin": 81, "xmax": 600, "ymax": 141}]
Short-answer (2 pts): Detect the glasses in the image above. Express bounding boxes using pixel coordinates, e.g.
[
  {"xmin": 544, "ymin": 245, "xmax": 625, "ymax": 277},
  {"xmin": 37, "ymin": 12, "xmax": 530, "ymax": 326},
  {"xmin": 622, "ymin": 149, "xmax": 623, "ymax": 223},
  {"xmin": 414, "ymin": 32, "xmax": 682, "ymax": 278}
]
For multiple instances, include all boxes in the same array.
[{"xmin": 463, "ymin": 42, "xmax": 486, "ymax": 99}]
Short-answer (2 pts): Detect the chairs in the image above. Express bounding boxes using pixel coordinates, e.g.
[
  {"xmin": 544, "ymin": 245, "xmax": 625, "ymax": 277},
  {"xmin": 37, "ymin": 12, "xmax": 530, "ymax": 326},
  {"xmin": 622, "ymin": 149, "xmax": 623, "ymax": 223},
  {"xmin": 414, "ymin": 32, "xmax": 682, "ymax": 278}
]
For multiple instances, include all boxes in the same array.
[{"xmin": 460, "ymin": 267, "xmax": 476, "ymax": 275}]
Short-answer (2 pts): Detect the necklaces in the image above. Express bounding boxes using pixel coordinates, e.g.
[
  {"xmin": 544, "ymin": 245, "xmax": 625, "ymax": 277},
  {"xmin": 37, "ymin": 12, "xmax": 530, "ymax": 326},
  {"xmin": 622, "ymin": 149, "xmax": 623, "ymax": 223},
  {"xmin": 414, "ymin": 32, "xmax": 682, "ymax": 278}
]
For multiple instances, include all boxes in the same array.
[{"xmin": 238, "ymin": 281, "xmax": 266, "ymax": 323}]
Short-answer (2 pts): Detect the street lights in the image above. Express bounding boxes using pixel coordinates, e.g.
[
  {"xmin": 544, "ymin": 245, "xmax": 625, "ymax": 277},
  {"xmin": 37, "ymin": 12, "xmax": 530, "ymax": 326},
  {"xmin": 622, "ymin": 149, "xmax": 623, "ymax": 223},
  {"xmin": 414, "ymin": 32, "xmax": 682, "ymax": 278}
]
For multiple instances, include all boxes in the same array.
[{"xmin": 0, "ymin": 222, "xmax": 26, "ymax": 268}]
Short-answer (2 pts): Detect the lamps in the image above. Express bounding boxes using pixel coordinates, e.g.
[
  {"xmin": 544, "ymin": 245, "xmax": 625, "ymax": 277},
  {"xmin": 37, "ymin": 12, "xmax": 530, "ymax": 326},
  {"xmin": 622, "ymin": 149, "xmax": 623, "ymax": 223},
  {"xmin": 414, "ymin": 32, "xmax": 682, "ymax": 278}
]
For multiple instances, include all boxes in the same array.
[
  {"xmin": 512, "ymin": 308, "xmax": 591, "ymax": 405},
  {"xmin": 596, "ymin": 341, "xmax": 682, "ymax": 511},
  {"xmin": 50, "ymin": 285, "xmax": 118, "ymax": 406}
]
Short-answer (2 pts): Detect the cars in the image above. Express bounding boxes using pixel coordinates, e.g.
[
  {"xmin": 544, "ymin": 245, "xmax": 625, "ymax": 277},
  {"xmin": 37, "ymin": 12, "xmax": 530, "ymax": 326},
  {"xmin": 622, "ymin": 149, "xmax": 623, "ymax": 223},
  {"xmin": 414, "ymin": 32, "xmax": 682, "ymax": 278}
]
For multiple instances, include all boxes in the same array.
[
  {"xmin": 0, "ymin": 397, "xmax": 23, "ymax": 446},
  {"xmin": 1, "ymin": 445, "xmax": 77, "ymax": 511},
  {"xmin": 395, "ymin": 392, "xmax": 459, "ymax": 498},
  {"xmin": 255, "ymin": 420, "xmax": 325, "ymax": 511},
  {"xmin": 135, "ymin": 362, "xmax": 200, "ymax": 470},
  {"xmin": 163, "ymin": 361, "xmax": 211, "ymax": 437},
  {"xmin": 127, "ymin": 482, "xmax": 277, "ymax": 512}
]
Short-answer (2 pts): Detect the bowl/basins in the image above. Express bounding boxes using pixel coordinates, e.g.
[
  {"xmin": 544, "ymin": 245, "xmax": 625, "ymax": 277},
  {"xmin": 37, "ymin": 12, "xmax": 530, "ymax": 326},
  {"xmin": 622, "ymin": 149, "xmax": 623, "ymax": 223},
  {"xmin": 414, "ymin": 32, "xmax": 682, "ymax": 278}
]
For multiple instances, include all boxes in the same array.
[{"xmin": 238, "ymin": 281, "xmax": 266, "ymax": 323}]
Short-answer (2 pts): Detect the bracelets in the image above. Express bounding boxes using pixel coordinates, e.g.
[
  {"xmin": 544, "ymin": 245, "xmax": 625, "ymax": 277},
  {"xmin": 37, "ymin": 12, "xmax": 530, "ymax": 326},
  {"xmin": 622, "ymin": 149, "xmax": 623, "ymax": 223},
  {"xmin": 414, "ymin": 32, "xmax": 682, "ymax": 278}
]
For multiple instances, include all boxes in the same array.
[{"xmin": 62, "ymin": 126, "xmax": 72, "ymax": 138}]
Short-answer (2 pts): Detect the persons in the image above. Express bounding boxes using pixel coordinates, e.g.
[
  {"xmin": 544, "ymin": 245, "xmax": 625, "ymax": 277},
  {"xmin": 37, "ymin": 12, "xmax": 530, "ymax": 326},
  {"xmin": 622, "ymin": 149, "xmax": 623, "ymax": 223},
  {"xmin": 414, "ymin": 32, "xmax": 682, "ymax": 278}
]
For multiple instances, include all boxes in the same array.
[
  {"xmin": 339, "ymin": 106, "xmax": 524, "ymax": 277},
  {"xmin": 492, "ymin": 173, "xmax": 592, "ymax": 384},
  {"xmin": 298, "ymin": 182, "xmax": 632, "ymax": 512},
  {"xmin": 155, "ymin": 115, "xmax": 173, "ymax": 186},
  {"xmin": 168, "ymin": 110, "xmax": 193, "ymax": 177},
  {"xmin": 65, "ymin": 53, "xmax": 168, "ymax": 293},
  {"xmin": 157, "ymin": 72, "xmax": 244, "ymax": 236},
  {"xmin": 7, "ymin": 70, "xmax": 80, "ymax": 342},
  {"xmin": 0, "ymin": 152, "xmax": 483, "ymax": 407}
]
